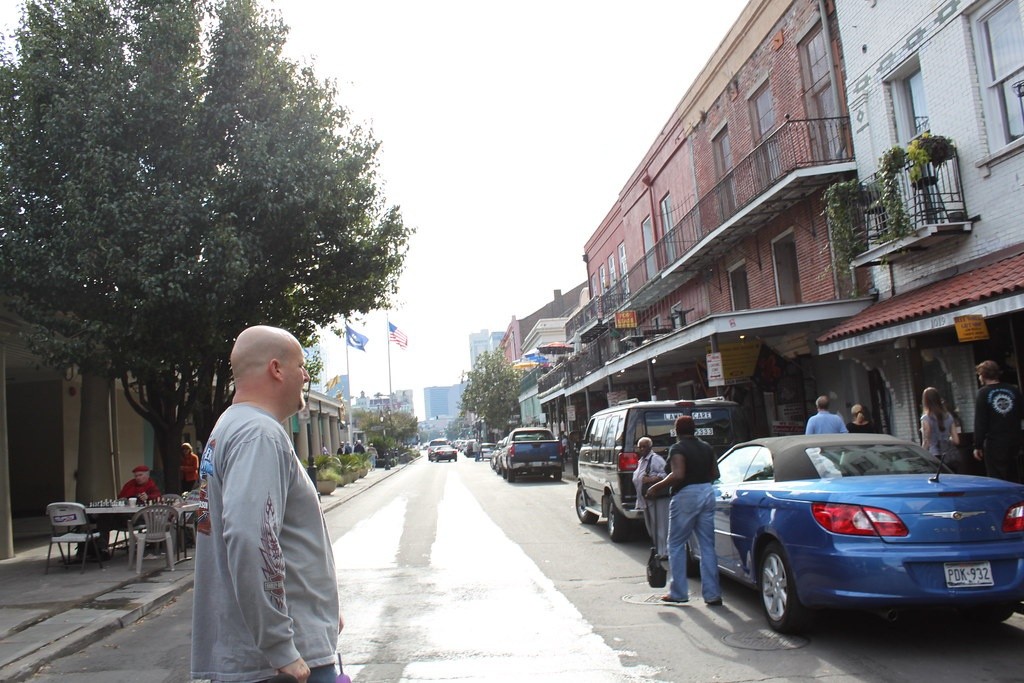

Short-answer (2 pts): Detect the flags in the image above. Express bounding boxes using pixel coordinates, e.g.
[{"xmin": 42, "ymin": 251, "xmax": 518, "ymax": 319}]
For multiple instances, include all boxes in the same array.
[
  {"xmin": 346, "ymin": 326, "xmax": 369, "ymax": 352},
  {"xmin": 389, "ymin": 322, "xmax": 407, "ymax": 351}
]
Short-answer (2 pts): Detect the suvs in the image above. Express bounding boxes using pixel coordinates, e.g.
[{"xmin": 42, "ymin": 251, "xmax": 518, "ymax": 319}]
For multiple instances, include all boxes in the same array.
[{"xmin": 569, "ymin": 395, "xmax": 753, "ymax": 544}]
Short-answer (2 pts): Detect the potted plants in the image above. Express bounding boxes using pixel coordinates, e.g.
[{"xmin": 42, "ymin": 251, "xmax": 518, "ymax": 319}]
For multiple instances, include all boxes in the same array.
[
  {"xmin": 817, "ymin": 134, "xmax": 955, "ymax": 299},
  {"xmin": 302, "ymin": 446, "xmax": 375, "ymax": 495}
]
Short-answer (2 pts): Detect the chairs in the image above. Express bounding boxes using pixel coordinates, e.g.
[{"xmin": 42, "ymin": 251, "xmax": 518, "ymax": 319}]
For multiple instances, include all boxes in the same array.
[{"xmin": 44, "ymin": 490, "xmax": 196, "ymax": 574}]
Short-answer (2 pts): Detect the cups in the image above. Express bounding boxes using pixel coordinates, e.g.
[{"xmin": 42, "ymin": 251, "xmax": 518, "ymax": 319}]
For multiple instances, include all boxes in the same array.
[{"xmin": 128, "ymin": 497, "xmax": 137, "ymax": 508}]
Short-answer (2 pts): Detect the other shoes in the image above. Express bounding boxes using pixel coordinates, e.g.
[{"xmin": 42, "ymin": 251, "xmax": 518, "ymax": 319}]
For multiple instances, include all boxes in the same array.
[
  {"xmin": 660, "ymin": 593, "xmax": 689, "ymax": 602},
  {"xmin": 88, "ymin": 550, "xmax": 111, "ymax": 562},
  {"xmin": 707, "ymin": 597, "xmax": 723, "ymax": 606}
]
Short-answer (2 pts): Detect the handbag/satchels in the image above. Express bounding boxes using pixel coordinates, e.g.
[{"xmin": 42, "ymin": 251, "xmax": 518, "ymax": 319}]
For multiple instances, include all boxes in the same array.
[
  {"xmin": 641, "ymin": 454, "xmax": 671, "ymax": 500},
  {"xmin": 646, "ymin": 547, "xmax": 666, "ymax": 588},
  {"xmin": 937, "ymin": 439, "xmax": 962, "ymax": 463}
]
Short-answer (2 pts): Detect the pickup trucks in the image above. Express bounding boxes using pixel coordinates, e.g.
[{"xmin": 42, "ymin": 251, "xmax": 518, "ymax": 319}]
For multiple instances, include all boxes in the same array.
[{"xmin": 499, "ymin": 427, "xmax": 566, "ymax": 483}]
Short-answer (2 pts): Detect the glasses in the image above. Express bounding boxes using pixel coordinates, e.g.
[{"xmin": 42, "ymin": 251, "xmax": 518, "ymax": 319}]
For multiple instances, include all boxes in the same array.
[{"xmin": 636, "ymin": 445, "xmax": 648, "ymax": 449}]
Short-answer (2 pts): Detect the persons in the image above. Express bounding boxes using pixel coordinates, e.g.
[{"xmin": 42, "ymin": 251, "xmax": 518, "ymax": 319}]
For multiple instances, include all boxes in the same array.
[
  {"xmin": 806, "ymin": 396, "xmax": 849, "ymax": 434},
  {"xmin": 846, "ymin": 404, "xmax": 879, "ymax": 433},
  {"xmin": 472, "ymin": 439, "xmax": 481, "ymax": 462},
  {"xmin": 558, "ymin": 431, "xmax": 581, "ymax": 477},
  {"xmin": 643, "ymin": 418, "xmax": 723, "ymax": 606},
  {"xmin": 920, "ymin": 387, "xmax": 959, "ymax": 459},
  {"xmin": 196, "ymin": 448, "xmax": 202, "ymax": 464},
  {"xmin": 337, "ymin": 440, "xmax": 365, "ymax": 454},
  {"xmin": 368, "ymin": 443, "xmax": 378, "ymax": 471},
  {"xmin": 179, "ymin": 442, "xmax": 199, "ymax": 492},
  {"xmin": 87, "ymin": 466, "xmax": 162, "ymax": 560},
  {"xmin": 632, "ymin": 437, "xmax": 670, "ymax": 560},
  {"xmin": 974, "ymin": 360, "xmax": 1024, "ymax": 484},
  {"xmin": 190, "ymin": 326, "xmax": 343, "ymax": 683}
]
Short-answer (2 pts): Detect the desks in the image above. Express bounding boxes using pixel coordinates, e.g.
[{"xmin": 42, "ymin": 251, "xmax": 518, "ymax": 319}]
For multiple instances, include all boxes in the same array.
[
  {"xmin": 83, "ymin": 502, "xmax": 200, "ymax": 565},
  {"xmin": 620, "ymin": 334, "xmax": 645, "ymax": 348}
]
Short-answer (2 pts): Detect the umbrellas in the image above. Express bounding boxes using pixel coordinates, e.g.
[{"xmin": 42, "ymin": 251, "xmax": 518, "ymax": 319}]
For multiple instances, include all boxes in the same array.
[{"xmin": 513, "ymin": 341, "xmax": 574, "ymax": 369}]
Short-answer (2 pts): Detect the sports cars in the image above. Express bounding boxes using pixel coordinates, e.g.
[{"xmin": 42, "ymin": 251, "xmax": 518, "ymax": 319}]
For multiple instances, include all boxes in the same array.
[{"xmin": 685, "ymin": 433, "xmax": 1024, "ymax": 637}]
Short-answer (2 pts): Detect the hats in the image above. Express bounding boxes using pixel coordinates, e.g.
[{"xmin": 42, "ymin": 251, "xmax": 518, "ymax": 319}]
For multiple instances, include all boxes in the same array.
[{"xmin": 132, "ymin": 465, "xmax": 148, "ymax": 472}]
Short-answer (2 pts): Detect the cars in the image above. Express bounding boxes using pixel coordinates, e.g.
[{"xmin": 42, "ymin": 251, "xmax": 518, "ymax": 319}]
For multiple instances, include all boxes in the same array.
[
  {"xmin": 429, "ymin": 444, "xmax": 457, "ymax": 462},
  {"xmin": 452, "ymin": 437, "xmax": 507, "ymax": 475},
  {"xmin": 420, "ymin": 437, "xmax": 451, "ymax": 454}
]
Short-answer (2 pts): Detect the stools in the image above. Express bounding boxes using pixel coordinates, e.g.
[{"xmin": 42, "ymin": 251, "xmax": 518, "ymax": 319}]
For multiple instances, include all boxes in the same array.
[
  {"xmin": 911, "ymin": 176, "xmax": 949, "ymax": 230},
  {"xmin": 864, "ymin": 206, "xmax": 889, "ymax": 252}
]
